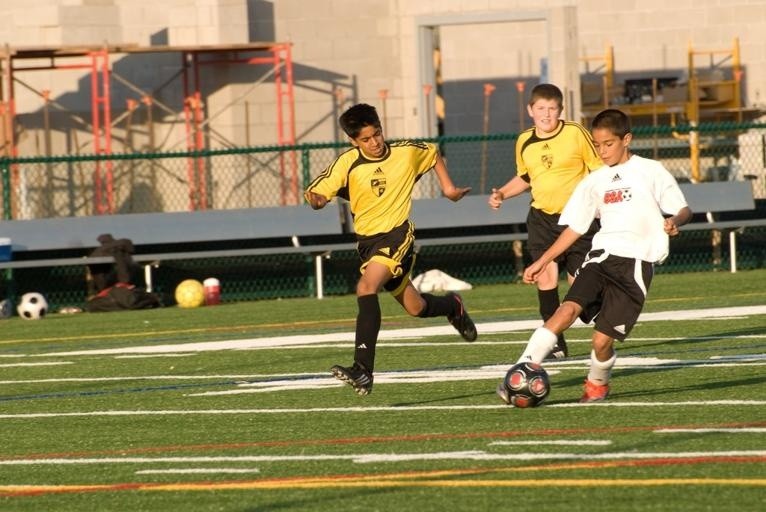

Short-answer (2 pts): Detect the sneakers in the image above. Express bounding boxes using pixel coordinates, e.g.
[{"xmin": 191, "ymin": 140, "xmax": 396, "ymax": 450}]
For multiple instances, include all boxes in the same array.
[
  {"xmin": 495, "ymin": 382, "xmax": 510, "ymax": 404},
  {"xmin": 542, "ymin": 344, "xmax": 570, "ymax": 361},
  {"xmin": 442, "ymin": 290, "xmax": 478, "ymax": 342},
  {"xmin": 578, "ymin": 380, "xmax": 611, "ymax": 404},
  {"xmin": 329, "ymin": 362, "xmax": 374, "ymax": 397}
]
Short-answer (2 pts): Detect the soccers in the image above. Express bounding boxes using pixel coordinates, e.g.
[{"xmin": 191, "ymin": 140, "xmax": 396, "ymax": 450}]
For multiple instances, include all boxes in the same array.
[
  {"xmin": 506, "ymin": 362, "xmax": 551, "ymax": 408},
  {"xmin": 176, "ymin": 279, "xmax": 204, "ymax": 307},
  {"xmin": 16, "ymin": 293, "xmax": 48, "ymax": 321}
]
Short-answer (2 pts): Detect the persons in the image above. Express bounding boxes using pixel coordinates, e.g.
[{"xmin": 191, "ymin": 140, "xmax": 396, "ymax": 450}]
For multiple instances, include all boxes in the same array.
[
  {"xmin": 305, "ymin": 103, "xmax": 476, "ymax": 396},
  {"xmin": 494, "ymin": 110, "xmax": 691, "ymax": 407},
  {"xmin": 488, "ymin": 85, "xmax": 607, "ymax": 360}
]
